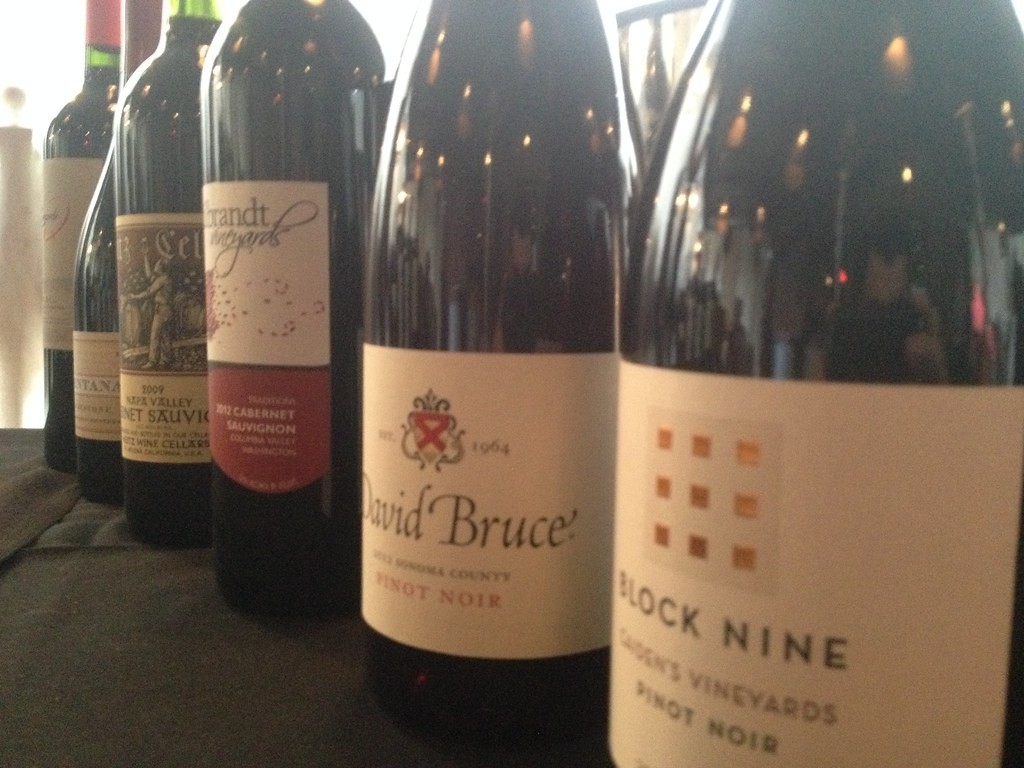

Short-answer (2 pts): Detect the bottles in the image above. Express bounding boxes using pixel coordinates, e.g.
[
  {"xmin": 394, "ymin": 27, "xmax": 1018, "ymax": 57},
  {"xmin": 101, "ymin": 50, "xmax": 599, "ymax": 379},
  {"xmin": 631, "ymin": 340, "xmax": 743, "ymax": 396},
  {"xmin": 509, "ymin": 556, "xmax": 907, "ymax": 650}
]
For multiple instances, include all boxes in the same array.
[
  {"xmin": 40, "ymin": 0, "xmax": 123, "ymax": 474},
  {"xmin": 355, "ymin": 0, "xmax": 625, "ymax": 749},
  {"xmin": 71, "ymin": 0, "xmax": 166, "ymax": 508},
  {"xmin": 112, "ymin": 0, "xmax": 224, "ymax": 550},
  {"xmin": 197, "ymin": 0, "xmax": 387, "ymax": 624},
  {"xmin": 600, "ymin": 0, "xmax": 1024, "ymax": 768}
]
